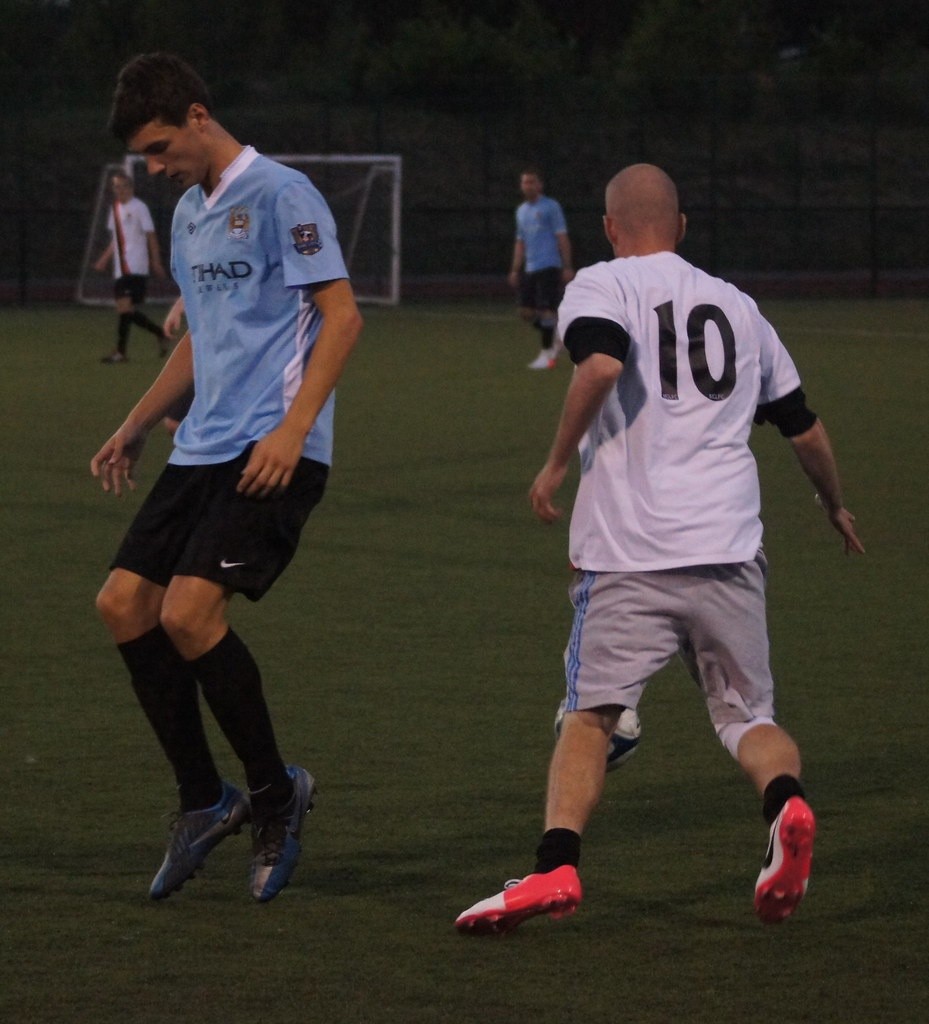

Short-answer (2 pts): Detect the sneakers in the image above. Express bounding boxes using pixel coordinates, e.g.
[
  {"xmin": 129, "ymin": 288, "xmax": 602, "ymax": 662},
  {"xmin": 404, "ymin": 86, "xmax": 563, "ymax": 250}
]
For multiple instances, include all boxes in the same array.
[
  {"xmin": 754, "ymin": 797, "xmax": 816, "ymax": 925},
  {"xmin": 454, "ymin": 864, "xmax": 582, "ymax": 937},
  {"xmin": 250, "ymin": 765, "xmax": 314, "ymax": 903},
  {"xmin": 148, "ymin": 781, "xmax": 250, "ymax": 901}
]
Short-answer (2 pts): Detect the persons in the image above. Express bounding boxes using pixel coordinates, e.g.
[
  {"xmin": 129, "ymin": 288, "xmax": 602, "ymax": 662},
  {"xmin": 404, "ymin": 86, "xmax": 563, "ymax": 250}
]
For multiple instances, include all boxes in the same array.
[
  {"xmin": 92, "ymin": 171, "xmax": 172, "ymax": 365},
  {"xmin": 455, "ymin": 161, "xmax": 868, "ymax": 935},
  {"xmin": 86, "ymin": 46, "xmax": 364, "ymax": 906},
  {"xmin": 505, "ymin": 168, "xmax": 575, "ymax": 373}
]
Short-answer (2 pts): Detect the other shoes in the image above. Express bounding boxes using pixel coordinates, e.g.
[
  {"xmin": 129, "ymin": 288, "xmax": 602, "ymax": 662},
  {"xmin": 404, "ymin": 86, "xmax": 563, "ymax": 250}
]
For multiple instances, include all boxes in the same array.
[
  {"xmin": 101, "ymin": 353, "xmax": 127, "ymax": 363},
  {"xmin": 159, "ymin": 337, "xmax": 170, "ymax": 357},
  {"xmin": 527, "ymin": 350, "xmax": 557, "ymax": 369}
]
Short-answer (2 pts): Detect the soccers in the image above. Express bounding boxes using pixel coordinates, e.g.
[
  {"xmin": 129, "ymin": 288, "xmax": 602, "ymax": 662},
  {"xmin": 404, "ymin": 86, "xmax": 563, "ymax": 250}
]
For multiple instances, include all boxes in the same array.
[{"xmin": 553, "ymin": 696, "xmax": 643, "ymax": 773}]
